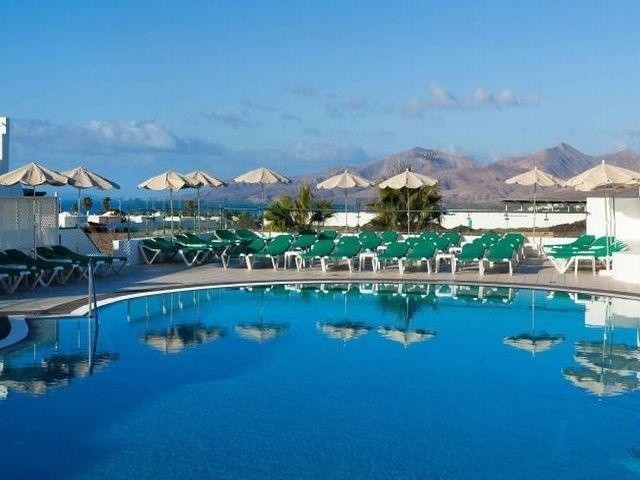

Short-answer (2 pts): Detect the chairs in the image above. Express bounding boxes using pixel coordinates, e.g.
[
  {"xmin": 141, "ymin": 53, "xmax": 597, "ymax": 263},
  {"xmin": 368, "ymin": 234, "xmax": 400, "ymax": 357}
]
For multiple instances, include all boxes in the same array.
[
  {"xmin": 242, "ymin": 282, "xmax": 601, "ymax": 309},
  {"xmin": 0, "ymin": 245, "xmax": 128, "ymax": 293},
  {"xmin": 136, "ymin": 231, "xmax": 525, "ymax": 277},
  {"xmin": 540, "ymin": 234, "xmax": 626, "ymax": 275}
]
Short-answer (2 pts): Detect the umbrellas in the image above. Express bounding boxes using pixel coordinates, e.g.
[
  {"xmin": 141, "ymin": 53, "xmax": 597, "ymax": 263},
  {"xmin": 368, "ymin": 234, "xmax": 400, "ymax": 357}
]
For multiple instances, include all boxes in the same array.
[
  {"xmin": 505, "ymin": 167, "xmax": 569, "ymax": 245},
  {"xmin": 313, "ymin": 170, "xmax": 376, "ymax": 236},
  {"xmin": 183, "ymin": 170, "xmax": 228, "ymax": 233},
  {"xmin": 562, "ymin": 162, "xmax": 639, "ymax": 271},
  {"xmin": 138, "ymin": 170, "xmax": 198, "ymax": 245},
  {"xmin": 379, "ymin": 169, "xmax": 438, "ymax": 235},
  {"xmin": 58, "ymin": 167, "xmax": 120, "ymax": 259},
  {"xmin": 2, "ymin": 161, "xmax": 73, "ymax": 260},
  {"xmin": 235, "ymin": 167, "xmax": 293, "ymax": 232}
]
